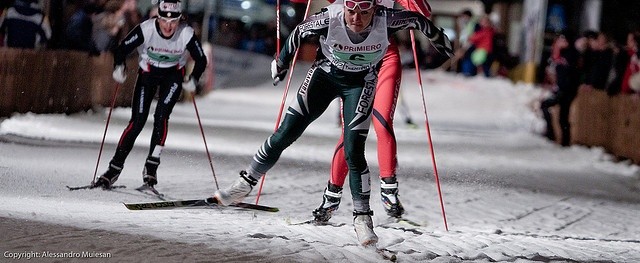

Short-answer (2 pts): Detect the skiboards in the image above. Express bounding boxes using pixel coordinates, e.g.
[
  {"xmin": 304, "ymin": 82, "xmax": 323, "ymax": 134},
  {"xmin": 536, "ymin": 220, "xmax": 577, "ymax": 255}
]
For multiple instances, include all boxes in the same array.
[
  {"xmin": 121, "ymin": 196, "xmax": 399, "ymax": 261},
  {"xmin": 64, "ymin": 182, "xmax": 177, "ymax": 202},
  {"xmin": 296, "ymin": 212, "xmax": 403, "ymax": 228}
]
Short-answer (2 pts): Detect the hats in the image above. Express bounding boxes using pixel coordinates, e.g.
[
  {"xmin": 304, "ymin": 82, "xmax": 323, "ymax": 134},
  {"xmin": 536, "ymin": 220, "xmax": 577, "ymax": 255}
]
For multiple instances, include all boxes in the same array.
[{"xmin": 157, "ymin": 0, "xmax": 182, "ymax": 21}]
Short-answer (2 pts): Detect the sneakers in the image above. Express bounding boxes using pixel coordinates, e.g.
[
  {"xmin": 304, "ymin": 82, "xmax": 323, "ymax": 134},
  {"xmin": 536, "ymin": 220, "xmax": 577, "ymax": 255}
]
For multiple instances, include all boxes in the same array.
[
  {"xmin": 313, "ymin": 180, "xmax": 343, "ymax": 221},
  {"xmin": 348, "ymin": 198, "xmax": 378, "ymax": 246},
  {"xmin": 97, "ymin": 163, "xmax": 124, "ymax": 185},
  {"xmin": 380, "ymin": 177, "xmax": 404, "ymax": 216},
  {"xmin": 142, "ymin": 159, "xmax": 160, "ymax": 184},
  {"xmin": 214, "ymin": 165, "xmax": 265, "ymax": 206}
]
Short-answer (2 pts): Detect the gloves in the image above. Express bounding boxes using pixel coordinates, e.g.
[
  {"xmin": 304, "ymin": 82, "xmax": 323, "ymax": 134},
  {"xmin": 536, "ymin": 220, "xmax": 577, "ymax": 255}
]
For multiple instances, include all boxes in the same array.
[
  {"xmin": 271, "ymin": 58, "xmax": 287, "ymax": 81},
  {"xmin": 429, "ymin": 27, "xmax": 455, "ymax": 59},
  {"xmin": 112, "ymin": 64, "xmax": 127, "ymax": 83},
  {"xmin": 182, "ymin": 77, "xmax": 196, "ymax": 92}
]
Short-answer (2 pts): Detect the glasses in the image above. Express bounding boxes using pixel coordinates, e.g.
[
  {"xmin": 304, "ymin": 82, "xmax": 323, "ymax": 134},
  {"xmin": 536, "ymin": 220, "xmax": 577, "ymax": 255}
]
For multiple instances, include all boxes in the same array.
[{"xmin": 344, "ymin": 0, "xmax": 373, "ymax": 11}]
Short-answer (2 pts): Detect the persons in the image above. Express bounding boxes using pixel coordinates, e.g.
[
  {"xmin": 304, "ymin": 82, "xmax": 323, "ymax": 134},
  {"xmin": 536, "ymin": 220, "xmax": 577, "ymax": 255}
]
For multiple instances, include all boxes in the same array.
[
  {"xmin": 412, "ymin": 8, "xmax": 520, "ymax": 76},
  {"xmin": 540, "ymin": 29, "xmax": 640, "ymax": 150},
  {"xmin": 214, "ymin": 0, "xmax": 455, "ymax": 244},
  {"xmin": 311, "ymin": 0, "xmax": 433, "ymax": 222},
  {"xmin": 189, "ymin": 11, "xmax": 292, "ymax": 57},
  {"xmin": 95, "ymin": 0, "xmax": 207, "ymax": 189},
  {"xmin": 0, "ymin": 0, "xmax": 142, "ymax": 53}
]
